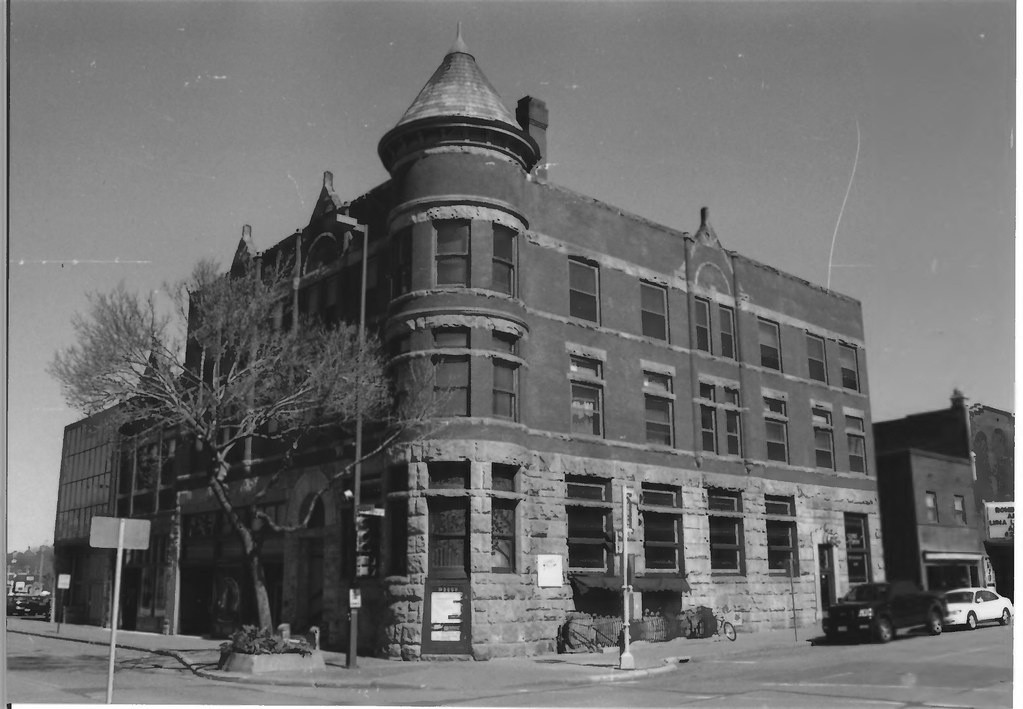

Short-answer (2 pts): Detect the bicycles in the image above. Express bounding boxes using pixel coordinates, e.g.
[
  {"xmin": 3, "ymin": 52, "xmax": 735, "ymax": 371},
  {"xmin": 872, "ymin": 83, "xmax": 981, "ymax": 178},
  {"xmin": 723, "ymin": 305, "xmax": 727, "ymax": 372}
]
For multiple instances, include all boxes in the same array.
[{"xmin": 697, "ymin": 606, "xmax": 736, "ymax": 642}]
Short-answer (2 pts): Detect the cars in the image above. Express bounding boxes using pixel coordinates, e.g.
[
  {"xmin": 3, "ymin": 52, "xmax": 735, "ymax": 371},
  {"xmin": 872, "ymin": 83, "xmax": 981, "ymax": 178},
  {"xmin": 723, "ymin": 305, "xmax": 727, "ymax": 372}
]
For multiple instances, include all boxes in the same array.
[
  {"xmin": 940, "ymin": 588, "xmax": 1014, "ymax": 631},
  {"xmin": 822, "ymin": 581, "xmax": 949, "ymax": 643}
]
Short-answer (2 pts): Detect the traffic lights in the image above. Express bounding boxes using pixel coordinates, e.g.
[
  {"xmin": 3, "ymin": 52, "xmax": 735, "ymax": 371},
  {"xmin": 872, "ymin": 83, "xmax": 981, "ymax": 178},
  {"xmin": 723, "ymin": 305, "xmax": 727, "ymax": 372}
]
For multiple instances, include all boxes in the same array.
[{"xmin": 621, "ymin": 484, "xmax": 643, "ymax": 534}]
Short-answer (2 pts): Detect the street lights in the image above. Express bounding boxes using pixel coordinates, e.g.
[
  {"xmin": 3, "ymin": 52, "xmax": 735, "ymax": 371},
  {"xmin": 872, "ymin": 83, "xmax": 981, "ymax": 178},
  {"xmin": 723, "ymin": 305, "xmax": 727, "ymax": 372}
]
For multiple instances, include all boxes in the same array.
[{"xmin": 337, "ymin": 201, "xmax": 365, "ymax": 670}]
